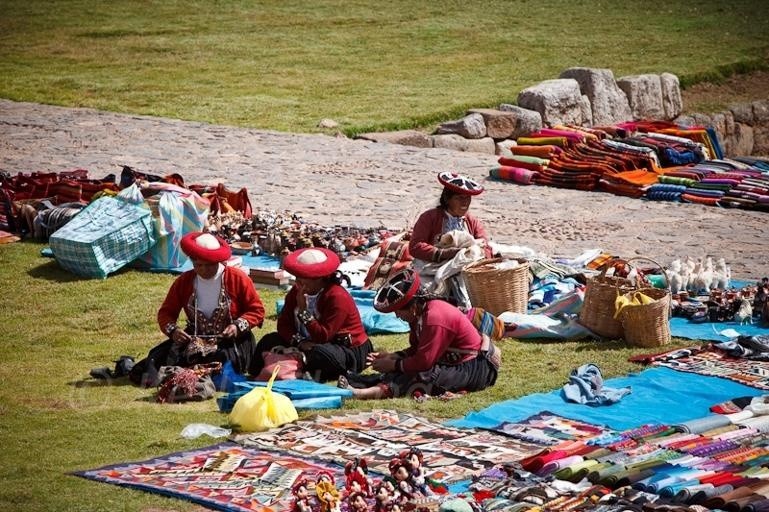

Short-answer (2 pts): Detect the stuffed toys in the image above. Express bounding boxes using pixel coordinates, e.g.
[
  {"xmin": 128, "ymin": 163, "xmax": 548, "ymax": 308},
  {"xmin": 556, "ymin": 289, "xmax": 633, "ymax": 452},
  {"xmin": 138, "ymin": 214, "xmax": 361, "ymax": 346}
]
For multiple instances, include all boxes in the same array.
[{"xmin": 662, "ymin": 256, "xmax": 769, "ymax": 323}]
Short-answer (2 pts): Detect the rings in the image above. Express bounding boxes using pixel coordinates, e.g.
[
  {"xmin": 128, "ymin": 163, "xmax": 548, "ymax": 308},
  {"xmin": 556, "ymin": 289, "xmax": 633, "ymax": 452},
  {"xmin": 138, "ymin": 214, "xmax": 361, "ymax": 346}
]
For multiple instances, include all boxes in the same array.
[{"xmin": 177, "ymin": 338, "xmax": 181, "ymax": 341}]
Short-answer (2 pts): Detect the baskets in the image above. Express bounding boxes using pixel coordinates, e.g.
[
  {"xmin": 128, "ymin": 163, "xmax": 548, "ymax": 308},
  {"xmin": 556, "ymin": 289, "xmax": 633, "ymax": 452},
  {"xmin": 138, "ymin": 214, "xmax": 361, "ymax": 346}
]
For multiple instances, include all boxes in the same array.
[
  {"xmin": 578, "ymin": 258, "xmax": 636, "ymax": 339},
  {"xmin": 462, "ymin": 255, "xmax": 529, "ymax": 316},
  {"xmin": 614, "ymin": 256, "xmax": 673, "ymax": 349}
]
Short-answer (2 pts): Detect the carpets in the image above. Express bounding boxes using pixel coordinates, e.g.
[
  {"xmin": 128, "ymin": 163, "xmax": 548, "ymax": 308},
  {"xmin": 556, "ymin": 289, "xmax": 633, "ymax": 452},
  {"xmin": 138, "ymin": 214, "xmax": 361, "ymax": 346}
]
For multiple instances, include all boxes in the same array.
[
  {"xmin": 442, "ymin": 367, "xmax": 764, "ymax": 454},
  {"xmin": 666, "ymin": 279, "xmax": 769, "ymax": 347},
  {"xmin": 165, "ymin": 244, "xmax": 282, "ymax": 272},
  {"xmin": 278, "ymin": 288, "xmax": 416, "ymax": 335},
  {"xmin": 72, "ymin": 408, "xmax": 551, "ymax": 507}
]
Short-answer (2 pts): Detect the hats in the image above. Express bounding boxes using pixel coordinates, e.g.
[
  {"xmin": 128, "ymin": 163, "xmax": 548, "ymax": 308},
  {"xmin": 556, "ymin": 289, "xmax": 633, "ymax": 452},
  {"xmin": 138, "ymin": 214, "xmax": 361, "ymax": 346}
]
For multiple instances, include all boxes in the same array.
[
  {"xmin": 348, "ymin": 492, "xmax": 366, "ymax": 503},
  {"xmin": 315, "ymin": 472, "xmax": 334, "ymax": 486},
  {"xmin": 346, "ymin": 473, "xmax": 368, "ymax": 491},
  {"xmin": 345, "ymin": 458, "xmax": 368, "ymax": 475},
  {"xmin": 388, "ymin": 458, "xmax": 412, "ymax": 476},
  {"xmin": 373, "ymin": 270, "xmax": 421, "ymax": 314},
  {"xmin": 316, "ymin": 482, "xmax": 340, "ymax": 511},
  {"xmin": 179, "ymin": 232, "xmax": 232, "ymax": 263},
  {"xmin": 400, "ymin": 446, "xmax": 424, "ymax": 468},
  {"xmin": 371, "ymin": 480, "xmax": 383, "ymax": 495},
  {"xmin": 438, "ymin": 172, "xmax": 483, "ymax": 195},
  {"xmin": 292, "ymin": 479, "xmax": 310, "ymax": 495},
  {"xmin": 283, "ymin": 247, "xmax": 340, "ymax": 280}
]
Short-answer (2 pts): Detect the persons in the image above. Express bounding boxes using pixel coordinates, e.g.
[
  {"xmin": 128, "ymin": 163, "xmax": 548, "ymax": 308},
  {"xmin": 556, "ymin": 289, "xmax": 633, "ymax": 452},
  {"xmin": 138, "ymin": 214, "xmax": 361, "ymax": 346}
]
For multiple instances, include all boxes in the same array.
[
  {"xmin": 291, "ymin": 446, "xmax": 431, "ymax": 511},
  {"xmin": 409, "ymin": 172, "xmax": 492, "ymax": 259},
  {"xmin": 204, "ymin": 209, "xmax": 398, "ymax": 260},
  {"xmin": 249, "ymin": 247, "xmax": 373, "ymax": 380},
  {"xmin": 130, "ymin": 231, "xmax": 265, "ymax": 389},
  {"xmin": 338, "ymin": 269, "xmax": 497, "ymax": 399}
]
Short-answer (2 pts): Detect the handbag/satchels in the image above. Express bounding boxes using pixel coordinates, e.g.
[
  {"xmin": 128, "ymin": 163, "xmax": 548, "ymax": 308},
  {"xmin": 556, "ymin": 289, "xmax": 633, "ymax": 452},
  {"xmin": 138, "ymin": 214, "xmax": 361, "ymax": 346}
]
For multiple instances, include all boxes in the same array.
[
  {"xmin": 48, "ymin": 193, "xmax": 156, "ymax": 276},
  {"xmin": 2, "ymin": 167, "xmax": 253, "ymax": 244},
  {"xmin": 419, "ymin": 333, "xmax": 503, "ymax": 371}
]
